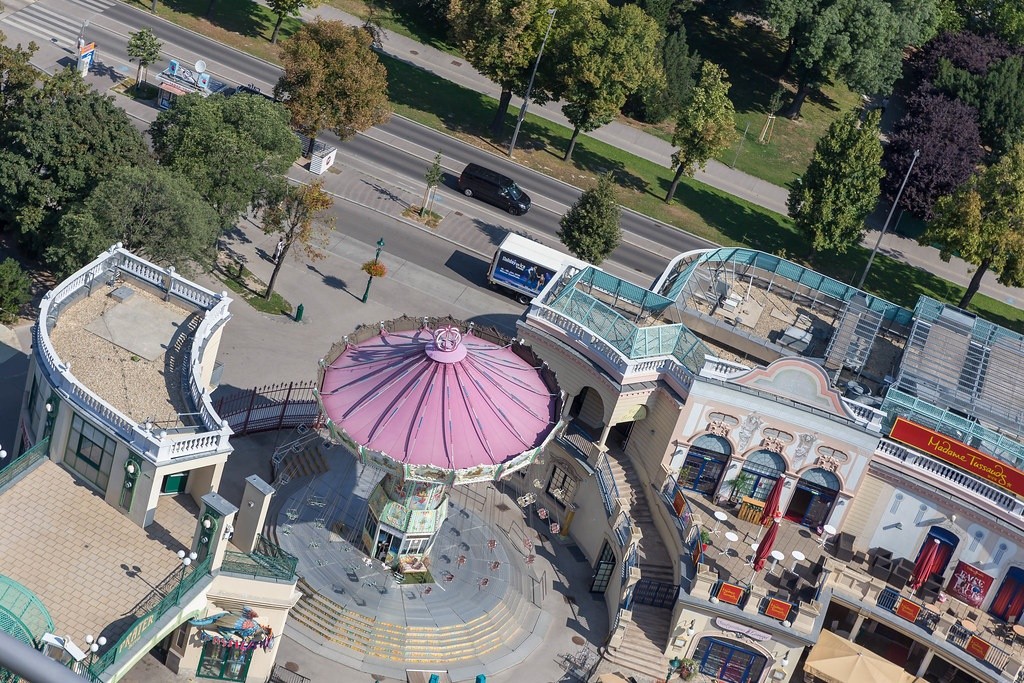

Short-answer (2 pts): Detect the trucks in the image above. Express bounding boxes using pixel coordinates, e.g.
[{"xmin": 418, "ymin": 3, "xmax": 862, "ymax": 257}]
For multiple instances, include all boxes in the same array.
[{"xmin": 487, "ymin": 232, "xmax": 605, "ymax": 305}]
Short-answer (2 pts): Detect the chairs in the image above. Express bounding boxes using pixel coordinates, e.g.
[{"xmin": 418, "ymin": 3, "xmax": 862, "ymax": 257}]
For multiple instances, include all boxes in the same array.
[{"xmin": 871, "ymin": 547, "xmax": 1024, "ymax": 646}]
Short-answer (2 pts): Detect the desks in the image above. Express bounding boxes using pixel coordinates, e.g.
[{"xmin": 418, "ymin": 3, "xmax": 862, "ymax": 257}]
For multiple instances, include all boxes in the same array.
[
  {"xmin": 925, "ymin": 604, "xmax": 941, "ymax": 615},
  {"xmin": 745, "ymin": 543, "xmax": 759, "ymax": 569},
  {"xmin": 707, "ymin": 511, "xmax": 728, "ymax": 537},
  {"xmin": 817, "ymin": 525, "xmax": 836, "ymax": 550},
  {"xmin": 961, "ymin": 620, "xmax": 976, "ymax": 631},
  {"xmin": 718, "ymin": 533, "xmax": 738, "ymax": 558},
  {"xmin": 854, "ymin": 550, "xmax": 866, "ymax": 564},
  {"xmin": 784, "ymin": 551, "xmax": 805, "ymax": 576},
  {"xmin": 767, "ymin": 550, "xmax": 783, "ymax": 578},
  {"xmin": 1013, "ymin": 625, "xmax": 1024, "ymax": 642}
]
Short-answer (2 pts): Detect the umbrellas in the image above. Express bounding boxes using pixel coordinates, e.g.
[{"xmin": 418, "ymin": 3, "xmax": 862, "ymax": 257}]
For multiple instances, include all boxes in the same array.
[
  {"xmin": 908, "ymin": 539, "xmax": 940, "ymax": 600},
  {"xmin": 803, "ymin": 628, "xmax": 930, "ymax": 683},
  {"xmin": 755, "ymin": 474, "xmax": 785, "ymax": 542},
  {"xmin": 748, "ymin": 518, "xmax": 780, "ymax": 584}
]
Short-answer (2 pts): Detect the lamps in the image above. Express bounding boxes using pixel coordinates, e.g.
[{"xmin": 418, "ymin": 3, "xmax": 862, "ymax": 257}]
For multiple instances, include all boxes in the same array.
[
  {"xmin": 782, "ymin": 650, "xmax": 790, "ymax": 667},
  {"xmin": 686, "ymin": 619, "xmax": 695, "ymax": 636}
]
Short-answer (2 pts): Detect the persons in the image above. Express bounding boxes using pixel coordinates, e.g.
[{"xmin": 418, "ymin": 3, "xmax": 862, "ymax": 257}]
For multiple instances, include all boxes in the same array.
[
  {"xmin": 273, "ymin": 238, "xmax": 283, "ymax": 261},
  {"xmin": 523, "ymin": 265, "xmax": 545, "ymax": 290}
]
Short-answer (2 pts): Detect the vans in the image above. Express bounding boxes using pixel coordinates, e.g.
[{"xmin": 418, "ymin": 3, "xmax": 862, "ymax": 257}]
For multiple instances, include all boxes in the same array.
[{"xmin": 458, "ymin": 162, "xmax": 532, "ymax": 216}]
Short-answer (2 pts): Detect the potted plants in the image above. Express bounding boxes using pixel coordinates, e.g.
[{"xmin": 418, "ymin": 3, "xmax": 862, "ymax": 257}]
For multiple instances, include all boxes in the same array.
[
  {"xmin": 728, "ymin": 473, "xmax": 754, "ymax": 507},
  {"xmin": 679, "ymin": 656, "xmax": 700, "ymax": 682},
  {"xmin": 676, "ymin": 464, "xmax": 692, "ymax": 488},
  {"xmin": 701, "ymin": 530, "xmax": 713, "ymax": 551}
]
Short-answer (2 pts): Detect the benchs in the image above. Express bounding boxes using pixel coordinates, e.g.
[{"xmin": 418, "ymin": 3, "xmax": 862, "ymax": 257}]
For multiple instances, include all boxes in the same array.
[
  {"xmin": 779, "ymin": 570, "xmax": 802, "ymax": 591},
  {"xmin": 834, "ymin": 531, "xmax": 856, "ymax": 562}
]
